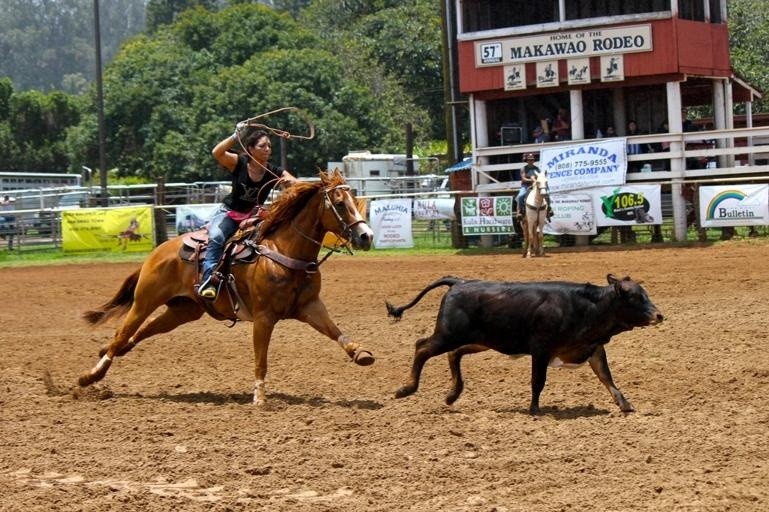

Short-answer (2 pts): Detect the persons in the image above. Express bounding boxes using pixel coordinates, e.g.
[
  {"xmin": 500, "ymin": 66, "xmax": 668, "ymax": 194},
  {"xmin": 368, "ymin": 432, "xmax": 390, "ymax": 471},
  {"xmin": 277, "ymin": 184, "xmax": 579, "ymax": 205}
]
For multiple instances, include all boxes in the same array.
[
  {"xmin": 553, "ymin": 105, "xmax": 571, "ymax": 140},
  {"xmin": 604, "ymin": 125, "xmax": 620, "ymax": 137},
  {"xmin": 0, "ymin": 194, "xmax": 18, "ymax": 251},
  {"xmin": 532, "ymin": 117, "xmax": 552, "ymax": 142},
  {"xmin": 196, "ymin": 119, "xmax": 298, "ymax": 299},
  {"xmin": 515, "ymin": 152, "xmax": 556, "ymax": 220},
  {"xmin": 624, "ymin": 118, "xmax": 649, "ymax": 173},
  {"xmin": 683, "ymin": 108, "xmax": 700, "ymax": 169},
  {"xmin": 653, "ymin": 119, "xmax": 671, "ymax": 171}
]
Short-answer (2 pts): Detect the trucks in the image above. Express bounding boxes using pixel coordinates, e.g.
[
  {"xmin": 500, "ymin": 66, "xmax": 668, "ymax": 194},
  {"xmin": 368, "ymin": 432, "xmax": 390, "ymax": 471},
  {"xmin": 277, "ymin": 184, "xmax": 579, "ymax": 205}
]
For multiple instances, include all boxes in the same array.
[{"xmin": 328, "ymin": 151, "xmax": 422, "ymax": 218}]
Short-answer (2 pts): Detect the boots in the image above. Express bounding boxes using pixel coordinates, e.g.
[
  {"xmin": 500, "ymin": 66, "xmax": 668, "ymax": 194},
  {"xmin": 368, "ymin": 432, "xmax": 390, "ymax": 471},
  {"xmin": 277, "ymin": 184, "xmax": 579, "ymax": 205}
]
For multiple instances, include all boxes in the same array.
[
  {"xmin": 546, "ymin": 208, "xmax": 553, "ymax": 218},
  {"xmin": 516, "ymin": 208, "xmax": 522, "ymax": 219}
]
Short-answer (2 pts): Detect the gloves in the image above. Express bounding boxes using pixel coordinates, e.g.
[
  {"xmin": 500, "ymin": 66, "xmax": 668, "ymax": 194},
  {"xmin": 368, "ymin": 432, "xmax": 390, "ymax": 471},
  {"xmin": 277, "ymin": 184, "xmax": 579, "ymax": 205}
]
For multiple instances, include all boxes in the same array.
[{"xmin": 231, "ymin": 122, "xmax": 250, "ymax": 144}]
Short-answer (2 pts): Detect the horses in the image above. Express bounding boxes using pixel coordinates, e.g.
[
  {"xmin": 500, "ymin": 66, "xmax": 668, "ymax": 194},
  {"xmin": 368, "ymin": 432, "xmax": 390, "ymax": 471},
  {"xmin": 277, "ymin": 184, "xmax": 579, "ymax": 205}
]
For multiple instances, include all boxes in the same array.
[
  {"xmin": 519, "ymin": 169, "xmax": 549, "ymax": 258},
  {"xmin": 76, "ymin": 166, "xmax": 375, "ymax": 406}
]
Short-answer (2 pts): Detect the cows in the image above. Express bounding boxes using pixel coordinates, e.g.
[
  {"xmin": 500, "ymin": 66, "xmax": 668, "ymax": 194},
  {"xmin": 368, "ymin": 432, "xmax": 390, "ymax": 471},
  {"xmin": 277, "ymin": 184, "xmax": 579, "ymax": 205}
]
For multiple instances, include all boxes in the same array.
[{"xmin": 383, "ymin": 273, "xmax": 664, "ymax": 418}]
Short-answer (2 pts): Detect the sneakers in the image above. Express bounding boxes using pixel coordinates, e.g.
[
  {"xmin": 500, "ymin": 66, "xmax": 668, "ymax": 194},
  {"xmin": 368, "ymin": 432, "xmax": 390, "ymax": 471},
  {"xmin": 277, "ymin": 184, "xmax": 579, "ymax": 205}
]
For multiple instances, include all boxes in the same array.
[{"xmin": 201, "ymin": 285, "xmax": 217, "ymax": 298}]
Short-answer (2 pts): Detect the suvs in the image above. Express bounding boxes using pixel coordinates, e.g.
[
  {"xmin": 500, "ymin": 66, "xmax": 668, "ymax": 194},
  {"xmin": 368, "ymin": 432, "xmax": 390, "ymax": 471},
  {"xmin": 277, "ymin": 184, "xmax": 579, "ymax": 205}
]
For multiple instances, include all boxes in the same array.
[{"xmin": 31, "ymin": 182, "xmax": 115, "ymax": 238}]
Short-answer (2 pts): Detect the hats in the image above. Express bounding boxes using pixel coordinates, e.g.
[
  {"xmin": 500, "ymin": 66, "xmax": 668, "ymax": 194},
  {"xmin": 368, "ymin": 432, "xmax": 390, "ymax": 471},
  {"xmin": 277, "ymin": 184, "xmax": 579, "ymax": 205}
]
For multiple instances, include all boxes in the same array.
[{"xmin": 526, "ymin": 154, "xmax": 534, "ymax": 160}]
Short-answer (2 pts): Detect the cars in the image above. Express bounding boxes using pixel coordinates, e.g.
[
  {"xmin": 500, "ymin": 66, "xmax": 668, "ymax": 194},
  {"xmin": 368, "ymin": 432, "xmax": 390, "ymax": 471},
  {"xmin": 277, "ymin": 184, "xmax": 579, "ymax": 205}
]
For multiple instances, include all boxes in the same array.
[{"xmin": 0, "ymin": 210, "xmax": 28, "ymax": 238}]
